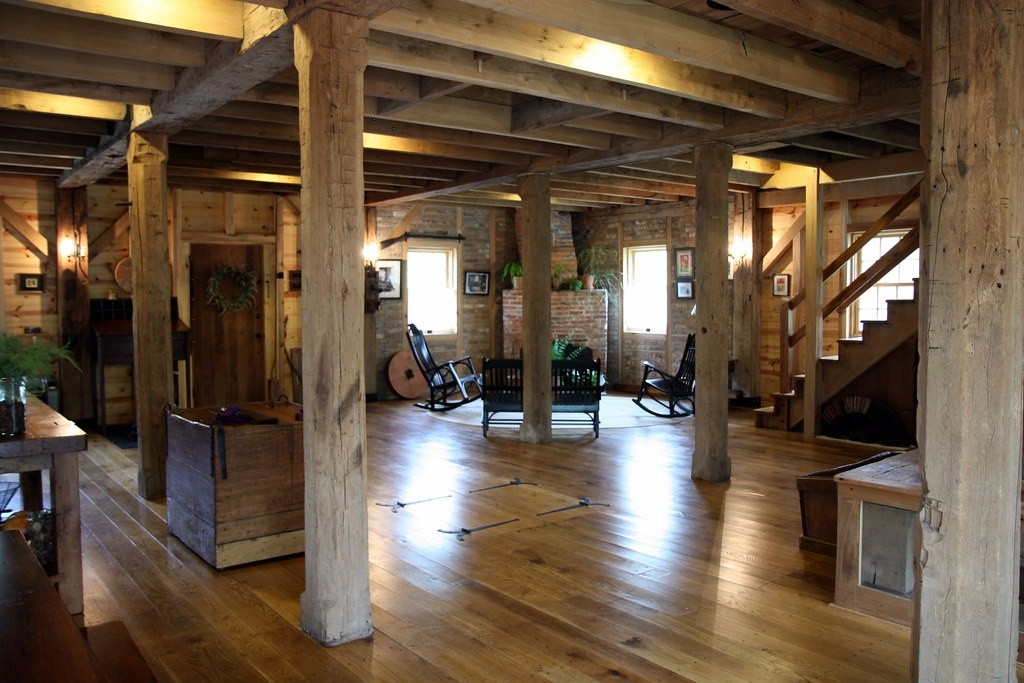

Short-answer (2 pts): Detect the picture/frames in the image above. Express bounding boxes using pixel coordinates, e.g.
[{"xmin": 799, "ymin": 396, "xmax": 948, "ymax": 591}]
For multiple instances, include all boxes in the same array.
[
  {"xmin": 676, "ymin": 280, "xmax": 694, "ymax": 299},
  {"xmin": 18, "ymin": 273, "xmax": 45, "ymax": 294},
  {"xmin": 376, "ymin": 259, "xmax": 402, "ymax": 300},
  {"xmin": 673, "ymin": 247, "xmax": 695, "ymax": 279},
  {"xmin": 464, "ymin": 271, "xmax": 490, "ymax": 295},
  {"xmin": 290, "ymin": 270, "xmax": 301, "ymax": 291},
  {"xmin": 773, "ymin": 274, "xmax": 789, "ymax": 297}
]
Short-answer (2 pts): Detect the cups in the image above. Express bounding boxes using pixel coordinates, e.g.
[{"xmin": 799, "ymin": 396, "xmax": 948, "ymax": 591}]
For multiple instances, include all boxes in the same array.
[{"xmin": 107, "ymin": 289, "xmax": 117, "ymax": 299}]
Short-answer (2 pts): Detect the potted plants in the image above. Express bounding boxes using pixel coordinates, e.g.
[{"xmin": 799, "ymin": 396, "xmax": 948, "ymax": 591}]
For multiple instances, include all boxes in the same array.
[
  {"xmin": 0, "ymin": 331, "xmax": 83, "ymax": 436},
  {"xmin": 575, "ymin": 247, "xmax": 623, "ymax": 307},
  {"xmin": 499, "ymin": 261, "xmax": 523, "ymax": 290},
  {"xmin": 552, "ymin": 261, "xmax": 577, "ymax": 291}
]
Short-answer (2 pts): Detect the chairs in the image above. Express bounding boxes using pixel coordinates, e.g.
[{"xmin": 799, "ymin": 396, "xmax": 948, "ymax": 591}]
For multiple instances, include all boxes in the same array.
[
  {"xmin": 406, "ymin": 324, "xmax": 482, "ymax": 412},
  {"xmin": 632, "ymin": 333, "xmax": 695, "ymax": 418}
]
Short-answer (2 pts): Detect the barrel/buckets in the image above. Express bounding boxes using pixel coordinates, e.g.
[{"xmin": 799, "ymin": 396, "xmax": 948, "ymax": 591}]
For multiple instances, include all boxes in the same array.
[{"xmin": 569, "ymin": 278, "xmax": 582, "ymax": 290}]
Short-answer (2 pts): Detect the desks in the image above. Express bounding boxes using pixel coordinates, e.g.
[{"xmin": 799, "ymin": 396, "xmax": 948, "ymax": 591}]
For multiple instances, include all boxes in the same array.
[{"xmin": 0, "ymin": 388, "xmax": 89, "ymax": 615}]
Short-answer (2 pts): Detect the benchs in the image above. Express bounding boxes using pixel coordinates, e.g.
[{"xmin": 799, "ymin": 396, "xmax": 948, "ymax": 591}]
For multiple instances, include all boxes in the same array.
[{"xmin": 477, "ymin": 357, "xmax": 606, "ymax": 438}]
